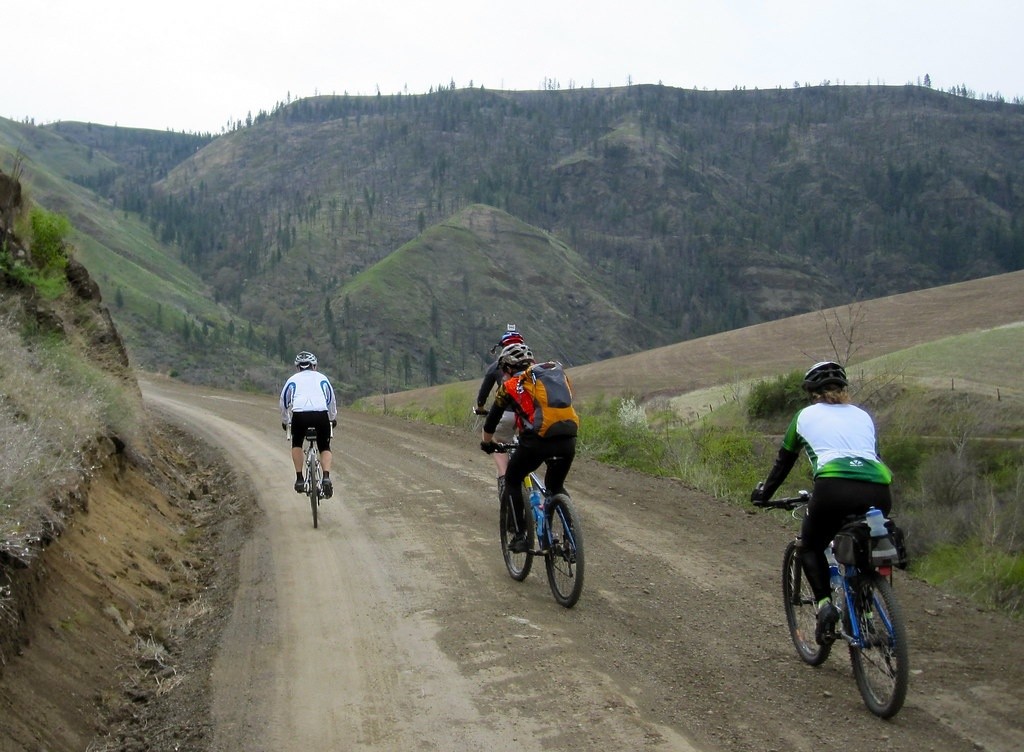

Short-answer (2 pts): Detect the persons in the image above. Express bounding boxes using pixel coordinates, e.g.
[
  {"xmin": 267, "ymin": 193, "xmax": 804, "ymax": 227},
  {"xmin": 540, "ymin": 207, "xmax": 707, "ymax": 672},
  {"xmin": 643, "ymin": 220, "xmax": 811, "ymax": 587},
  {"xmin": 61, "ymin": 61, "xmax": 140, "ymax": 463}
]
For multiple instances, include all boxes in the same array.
[
  {"xmin": 751, "ymin": 361, "xmax": 893, "ymax": 645},
  {"xmin": 477, "ymin": 330, "xmax": 579, "ymax": 565},
  {"xmin": 280, "ymin": 351, "xmax": 337, "ymax": 499}
]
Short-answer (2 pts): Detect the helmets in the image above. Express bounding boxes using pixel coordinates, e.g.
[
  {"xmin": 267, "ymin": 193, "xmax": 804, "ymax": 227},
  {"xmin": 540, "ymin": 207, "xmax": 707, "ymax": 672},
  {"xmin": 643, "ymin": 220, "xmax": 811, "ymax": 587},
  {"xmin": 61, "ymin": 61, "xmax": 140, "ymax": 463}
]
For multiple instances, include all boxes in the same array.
[
  {"xmin": 497, "ymin": 343, "xmax": 534, "ymax": 362},
  {"xmin": 295, "ymin": 351, "xmax": 317, "ymax": 366},
  {"xmin": 802, "ymin": 361, "xmax": 848, "ymax": 388},
  {"xmin": 500, "ymin": 331, "xmax": 524, "ymax": 344}
]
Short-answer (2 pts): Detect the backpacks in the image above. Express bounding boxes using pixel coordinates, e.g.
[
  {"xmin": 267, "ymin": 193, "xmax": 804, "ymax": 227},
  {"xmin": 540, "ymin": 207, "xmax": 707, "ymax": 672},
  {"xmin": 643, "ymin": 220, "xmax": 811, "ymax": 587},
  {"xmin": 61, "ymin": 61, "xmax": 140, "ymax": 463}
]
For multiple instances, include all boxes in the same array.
[{"xmin": 514, "ymin": 361, "xmax": 579, "ymax": 437}]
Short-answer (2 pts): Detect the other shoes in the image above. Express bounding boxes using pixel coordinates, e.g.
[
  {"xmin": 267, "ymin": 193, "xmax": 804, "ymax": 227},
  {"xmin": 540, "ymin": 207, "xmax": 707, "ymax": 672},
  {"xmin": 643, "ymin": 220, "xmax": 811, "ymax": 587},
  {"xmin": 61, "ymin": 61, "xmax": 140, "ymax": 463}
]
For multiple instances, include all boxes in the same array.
[{"xmin": 508, "ymin": 535, "xmax": 530, "ymax": 554}]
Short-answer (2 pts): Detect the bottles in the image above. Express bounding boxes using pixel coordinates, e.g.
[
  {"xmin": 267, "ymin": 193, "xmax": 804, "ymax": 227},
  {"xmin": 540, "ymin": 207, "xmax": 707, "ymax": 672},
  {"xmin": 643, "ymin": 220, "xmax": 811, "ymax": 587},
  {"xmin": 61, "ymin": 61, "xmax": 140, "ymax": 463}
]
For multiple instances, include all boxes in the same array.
[
  {"xmin": 824, "ymin": 546, "xmax": 842, "ymax": 590},
  {"xmin": 867, "ymin": 507, "xmax": 889, "ymax": 537},
  {"xmin": 537, "ymin": 505, "xmax": 544, "ymax": 536},
  {"xmin": 529, "ymin": 490, "xmax": 542, "ymax": 519}
]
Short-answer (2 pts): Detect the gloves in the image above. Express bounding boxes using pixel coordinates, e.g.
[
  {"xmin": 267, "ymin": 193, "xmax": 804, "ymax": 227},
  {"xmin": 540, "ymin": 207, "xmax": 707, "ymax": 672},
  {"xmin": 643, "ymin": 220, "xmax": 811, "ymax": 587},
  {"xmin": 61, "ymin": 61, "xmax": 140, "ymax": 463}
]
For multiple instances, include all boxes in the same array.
[
  {"xmin": 281, "ymin": 422, "xmax": 287, "ymax": 431},
  {"xmin": 750, "ymin": 481, "xmax": 765, "ymax": 501},
  {"xmin": 330, "ymin": 419, "xmax": 337, "ymax": 428},
  {"xmin": 481, "ymin": 441, "xmax": 503, "ymax": 454}
]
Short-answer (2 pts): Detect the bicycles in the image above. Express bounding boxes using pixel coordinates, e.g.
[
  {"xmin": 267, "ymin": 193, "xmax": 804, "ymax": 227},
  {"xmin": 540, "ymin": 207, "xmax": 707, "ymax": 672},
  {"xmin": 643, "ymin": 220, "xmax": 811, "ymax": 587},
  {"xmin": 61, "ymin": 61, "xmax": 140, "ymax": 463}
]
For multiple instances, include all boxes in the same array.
[
  {"xmin": 471, "ymin": 406, "xmax": 585, "ymax": 607},
  {"xmin": 285, "ymin": 422, "xmax": 332, "ymax": 529},
  {"xmin": 752, "ymin": 481, "xmax": 909, "ymax": 719}
]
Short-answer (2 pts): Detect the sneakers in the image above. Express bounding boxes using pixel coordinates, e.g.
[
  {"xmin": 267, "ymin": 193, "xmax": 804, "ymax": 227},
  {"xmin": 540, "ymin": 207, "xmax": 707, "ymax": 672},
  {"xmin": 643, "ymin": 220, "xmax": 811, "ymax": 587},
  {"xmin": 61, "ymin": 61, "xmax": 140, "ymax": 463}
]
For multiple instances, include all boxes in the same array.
[
  {"xmin": 322, "ymin": 479, "xmax": 334, "ymax": 498},
  {"xmin": 815, "ymin": 602, "xmax": 839, "ymax": 645},
  {"xmin": 294, "ymin": 480, "xmax": 305, "ymax": 493}
]
